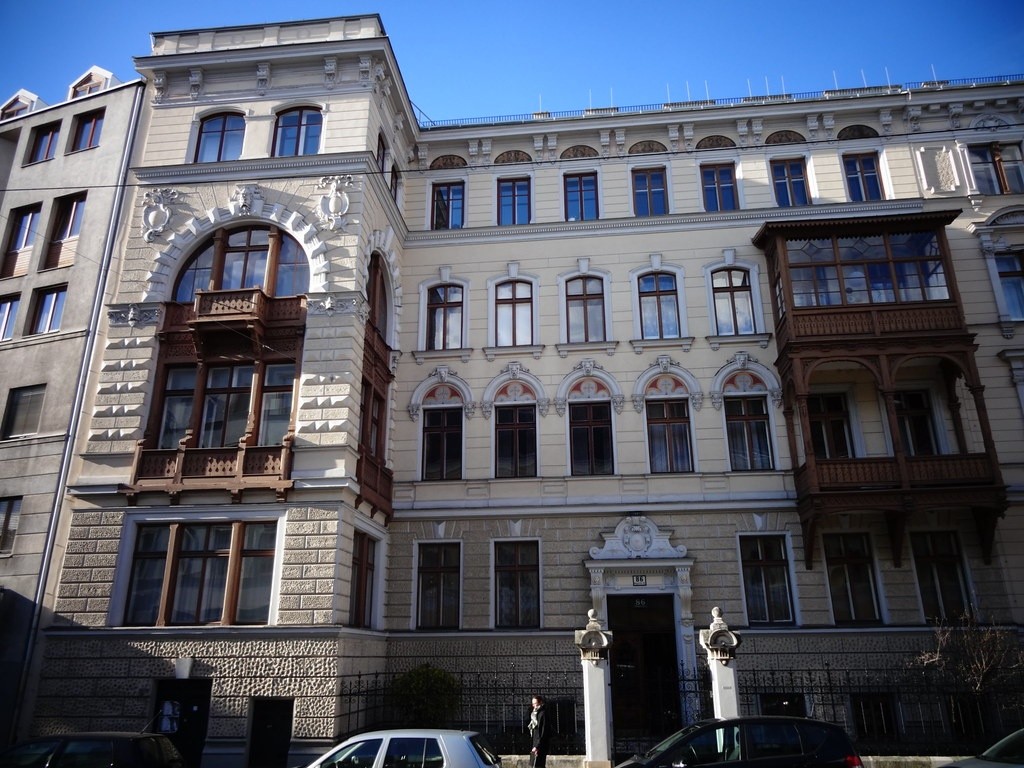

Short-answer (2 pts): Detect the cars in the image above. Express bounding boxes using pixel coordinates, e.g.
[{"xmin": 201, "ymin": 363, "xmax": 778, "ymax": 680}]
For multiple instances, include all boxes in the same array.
[
  {"xmin": 288, "ymin": 727, "xmax": 504, "ymax": 768},
  {"xmin": 613, "ymin": 713, "xmax": 865, "ymax": 768},
  {"xmin": 934, "ymin": 727, "xmax": 1024, "ymax": 768},
  {"xmin": 0, "ymin": 731, "xmax": 187, "ymax": 768}
]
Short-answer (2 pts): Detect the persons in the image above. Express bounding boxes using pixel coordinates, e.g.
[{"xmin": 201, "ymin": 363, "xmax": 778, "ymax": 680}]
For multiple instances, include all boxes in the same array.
[{"xmin": 528, "ymin": 696, "xmax": 550, "ymax": 768}]
